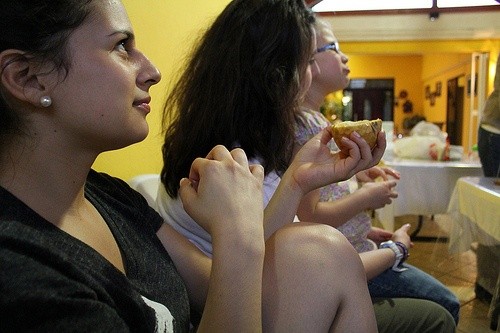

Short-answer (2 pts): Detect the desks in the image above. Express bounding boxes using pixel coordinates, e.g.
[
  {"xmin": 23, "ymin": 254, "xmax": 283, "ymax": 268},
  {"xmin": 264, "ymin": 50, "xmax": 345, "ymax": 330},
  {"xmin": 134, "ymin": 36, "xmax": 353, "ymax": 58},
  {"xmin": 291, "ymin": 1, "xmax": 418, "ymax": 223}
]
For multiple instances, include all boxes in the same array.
[
  {"xmin": 451, "ymin": 178, "xmax": 500, "ymax": 333},
  {"xmin": 376, "ymin": 144, "xmax": 485, "ymax": 243}
]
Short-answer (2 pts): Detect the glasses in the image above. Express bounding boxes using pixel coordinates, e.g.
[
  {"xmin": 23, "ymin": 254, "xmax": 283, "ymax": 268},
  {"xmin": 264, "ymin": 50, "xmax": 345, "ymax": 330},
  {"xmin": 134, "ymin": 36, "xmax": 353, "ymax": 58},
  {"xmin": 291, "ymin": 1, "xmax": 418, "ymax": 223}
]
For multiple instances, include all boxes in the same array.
[{"xmin": 316, "ymin": 41, "xmax": 340, "ymax": 53}]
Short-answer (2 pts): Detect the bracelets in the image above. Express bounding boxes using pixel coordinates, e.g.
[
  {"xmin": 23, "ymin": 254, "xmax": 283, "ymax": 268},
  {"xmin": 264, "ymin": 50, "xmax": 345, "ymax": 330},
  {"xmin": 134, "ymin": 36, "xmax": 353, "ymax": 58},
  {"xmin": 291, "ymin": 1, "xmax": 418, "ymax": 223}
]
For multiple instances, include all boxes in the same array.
[{"xmin": 394, "ymin": 241, "xmax": 407, "ymax": 266}]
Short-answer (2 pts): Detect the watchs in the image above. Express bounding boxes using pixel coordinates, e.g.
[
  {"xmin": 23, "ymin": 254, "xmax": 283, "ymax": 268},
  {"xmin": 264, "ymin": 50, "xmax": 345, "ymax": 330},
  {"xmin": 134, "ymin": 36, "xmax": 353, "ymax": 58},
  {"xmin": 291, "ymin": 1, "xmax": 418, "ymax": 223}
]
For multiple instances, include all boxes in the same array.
[{"xmin": 375, "ymin": 238, "xmax": 409, "ymax": 272}]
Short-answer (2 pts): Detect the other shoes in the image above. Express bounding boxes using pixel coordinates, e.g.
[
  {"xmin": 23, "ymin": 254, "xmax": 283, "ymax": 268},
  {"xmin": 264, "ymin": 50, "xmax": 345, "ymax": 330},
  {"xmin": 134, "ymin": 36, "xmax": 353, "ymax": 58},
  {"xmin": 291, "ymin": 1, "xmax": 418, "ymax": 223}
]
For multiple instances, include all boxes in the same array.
[{"xmin": 475, "ymin": 282, "xmax": 493, "ymax": 304}]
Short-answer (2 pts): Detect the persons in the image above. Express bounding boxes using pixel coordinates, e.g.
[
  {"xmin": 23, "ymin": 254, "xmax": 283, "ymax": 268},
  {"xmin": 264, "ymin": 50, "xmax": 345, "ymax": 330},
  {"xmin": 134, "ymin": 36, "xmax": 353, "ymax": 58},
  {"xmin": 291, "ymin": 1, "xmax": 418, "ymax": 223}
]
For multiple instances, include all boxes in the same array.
[
  {"xmin": 290, "ymin": 17, "xmax": 461, "ymax": 327},
  {"xmin": 478, "ymin": 53, "xmax": 500, "ymax": 177},
  {"xmin": 157, "ymin": 0, "xmax": 459, "ymax": 333},
  {"xmin": 0, "ymin": 0, "xmax": 387, "ymax": 333}
]
